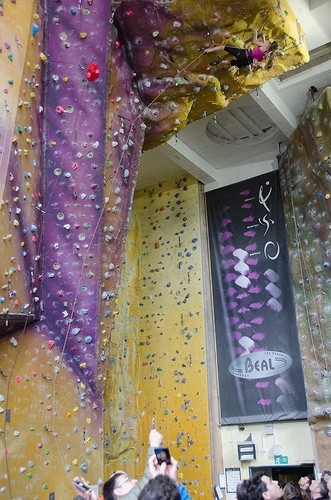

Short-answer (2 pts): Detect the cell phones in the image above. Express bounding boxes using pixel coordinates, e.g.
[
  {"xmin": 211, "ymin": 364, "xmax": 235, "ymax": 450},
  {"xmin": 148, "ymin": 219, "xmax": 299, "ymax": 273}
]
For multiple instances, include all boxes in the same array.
[
  {"xmin": 152, "ymin": 418, "xmax": 155, "ymax": 429},
  {"xmin": 154, "ymin": 447, "xmax": 171, "ymax": 465}
]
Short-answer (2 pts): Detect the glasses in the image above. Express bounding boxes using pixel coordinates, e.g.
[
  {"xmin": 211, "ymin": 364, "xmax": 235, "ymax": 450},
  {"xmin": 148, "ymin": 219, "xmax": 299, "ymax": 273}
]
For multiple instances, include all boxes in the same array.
[{"xmin": 118, "ymin": 477, "xmax": 131, "ymax": 486}]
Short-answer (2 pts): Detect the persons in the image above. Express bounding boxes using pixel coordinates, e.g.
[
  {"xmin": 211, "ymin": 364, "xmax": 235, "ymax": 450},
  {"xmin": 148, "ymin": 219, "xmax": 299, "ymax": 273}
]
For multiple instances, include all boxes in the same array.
[
  {"xmin": 201, "ymin": 30, "xmax": 280, "ymax": 71},
  {"xmin": 72, "ymin": 429, "xmax": 192, "ymax": 499},
  {"xmin": 235, "ymin": 469, "xmax": 331, "ymax": 499}
]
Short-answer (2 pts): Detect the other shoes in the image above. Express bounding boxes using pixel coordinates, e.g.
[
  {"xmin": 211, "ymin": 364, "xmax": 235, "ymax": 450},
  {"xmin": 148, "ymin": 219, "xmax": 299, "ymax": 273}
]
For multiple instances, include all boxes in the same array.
[
  {"xmin": 200, "ymin": 48, "xmax": 207, "ymax": 56},
  {"xmin": 210, "ymin": 60, "xmax": 219, "ymax": 66}
]
